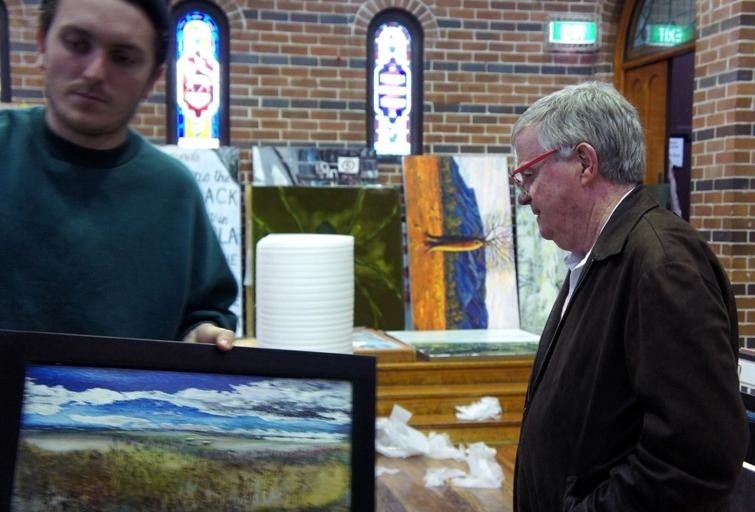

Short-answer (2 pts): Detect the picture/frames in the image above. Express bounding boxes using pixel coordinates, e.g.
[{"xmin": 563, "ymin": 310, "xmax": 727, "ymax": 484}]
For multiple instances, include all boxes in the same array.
[
  {"xmin": 0, "ymin": 327, "xmax": 375, "ymax": 511},
  {"xmin": 235, "ymin": 328, "xmax": 417, "ymax": 363}
]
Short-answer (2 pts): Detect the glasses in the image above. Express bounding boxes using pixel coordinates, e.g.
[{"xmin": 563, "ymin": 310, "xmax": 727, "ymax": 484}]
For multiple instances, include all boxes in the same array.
[{"xmin": 509, "ymin": 148, "xmax": 560, "ymax": 192}]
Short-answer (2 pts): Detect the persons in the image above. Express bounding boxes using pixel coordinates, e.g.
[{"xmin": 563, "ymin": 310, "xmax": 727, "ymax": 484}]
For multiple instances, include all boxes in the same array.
[
  {"xmin": 0, "ymin": 0, "xmax": 240, "ymax": 352},
  {"xmin": 508, "ymin": 81, "xmax": 749, "ymax": 511}
]
